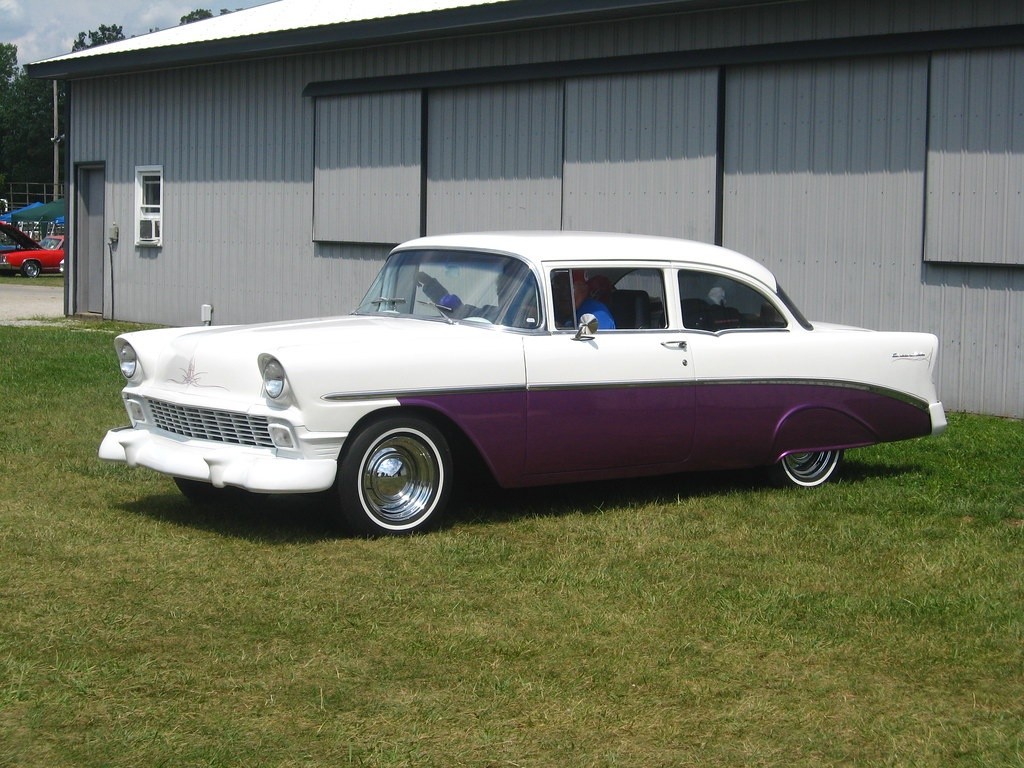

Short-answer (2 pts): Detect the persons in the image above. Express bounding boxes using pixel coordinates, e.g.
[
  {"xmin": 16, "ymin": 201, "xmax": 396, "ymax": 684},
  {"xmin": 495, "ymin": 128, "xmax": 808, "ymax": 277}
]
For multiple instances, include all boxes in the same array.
[{"xmin": 414, "ymin": 258, "xmax": 616, "ymax": 329}]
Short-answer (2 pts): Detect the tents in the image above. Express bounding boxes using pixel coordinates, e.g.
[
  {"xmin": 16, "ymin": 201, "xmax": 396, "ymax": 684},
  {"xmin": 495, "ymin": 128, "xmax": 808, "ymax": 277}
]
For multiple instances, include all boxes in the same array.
[{"xmin": 0, "ymin": 197, "xmax": 66, "ymax": 240}]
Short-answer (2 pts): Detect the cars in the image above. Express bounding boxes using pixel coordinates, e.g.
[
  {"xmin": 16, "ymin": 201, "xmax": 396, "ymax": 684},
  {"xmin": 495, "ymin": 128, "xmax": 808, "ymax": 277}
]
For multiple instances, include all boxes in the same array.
[
  {"xmin": 97, "ymin": 228, "xmax": 948, "ymax": 535},
  {"xmin": 0, "ymin": 220, "xmax": 64, "ymax": 279}
]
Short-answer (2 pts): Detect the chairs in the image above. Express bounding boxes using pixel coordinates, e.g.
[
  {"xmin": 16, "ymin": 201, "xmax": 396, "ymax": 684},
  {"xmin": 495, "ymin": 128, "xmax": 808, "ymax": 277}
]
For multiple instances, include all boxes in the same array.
[{"xmin": 608, "ymin": 290, "xmax": 651, "ymax": 329}]
